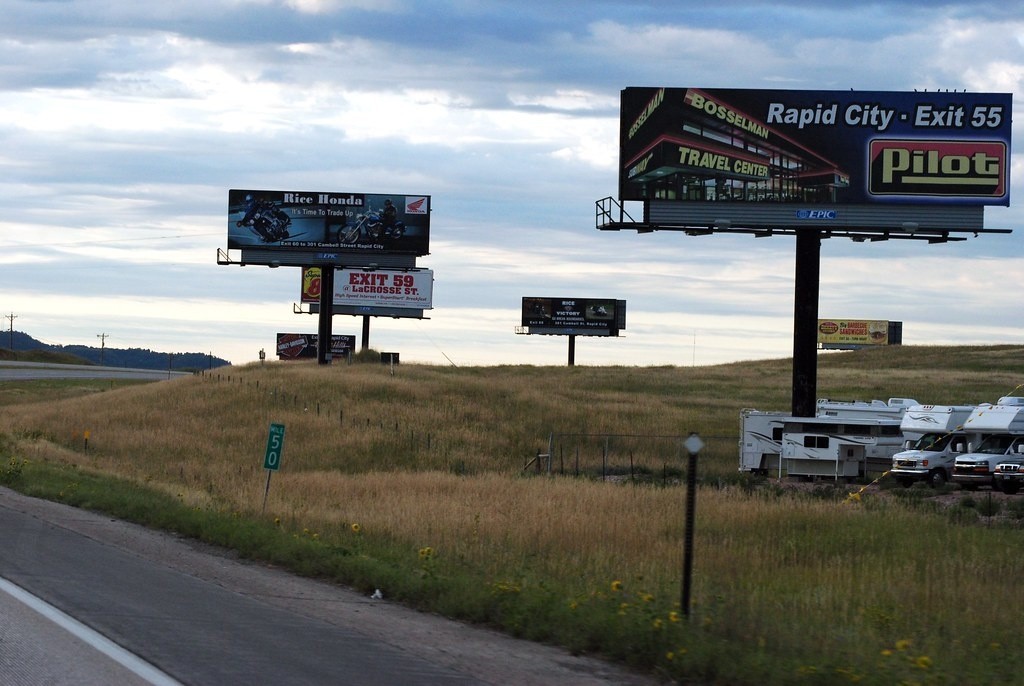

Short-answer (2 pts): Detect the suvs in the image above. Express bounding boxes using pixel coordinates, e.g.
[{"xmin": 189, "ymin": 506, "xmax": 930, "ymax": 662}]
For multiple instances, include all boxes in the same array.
[{"xmin": 992, "ymin": 436, "xmax": 1024, "ymax": 494}]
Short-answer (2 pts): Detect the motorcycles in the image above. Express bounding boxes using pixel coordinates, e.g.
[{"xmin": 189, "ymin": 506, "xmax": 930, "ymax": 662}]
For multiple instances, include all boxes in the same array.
[
  {"xmin": 235, "ymin": 199, "xmax": 292, "ymax": 241},
  {"xmin": 336, "ymin": 201, "xmax": 408, "ymax": 246}
]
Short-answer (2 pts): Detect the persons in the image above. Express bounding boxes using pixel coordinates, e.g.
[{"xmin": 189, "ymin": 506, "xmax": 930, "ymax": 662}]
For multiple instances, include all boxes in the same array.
[
  {"xmin": 239, "ymin": 194, "xmax": 288, "ymax": 242},
  {"xmin": 593, "ymin": 304, "xmax": 599, "ymax": 315},
  {"xmin": 523, "ymin": 298, "xmax": 546, "ymax": 317},
  {"xmin": 376, "ymin": 199, "xmax": 397, "ymax": 245},
  {"xmin": 708, "ymin": 190, "xmax": 802, "ymax": 201}
]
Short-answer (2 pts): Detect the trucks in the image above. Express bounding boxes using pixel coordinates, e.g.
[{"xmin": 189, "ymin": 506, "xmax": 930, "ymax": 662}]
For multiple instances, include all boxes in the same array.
[
  {"xmin": 738, "ymin": 397, "xmax": 921, "ymax": 486},
  {"xmin": 889, "ymin": 403, "xmax": 976, "ymax": 489},
  {"xmin": 953, "ymin": 394, "xmax": 1024, "ymax": 490}
]
